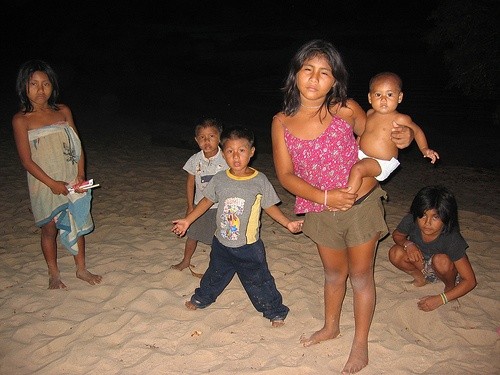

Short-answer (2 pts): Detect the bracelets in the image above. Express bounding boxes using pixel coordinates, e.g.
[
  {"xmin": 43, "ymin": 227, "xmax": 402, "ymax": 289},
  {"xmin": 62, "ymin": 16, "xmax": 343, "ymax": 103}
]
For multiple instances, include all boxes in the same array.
[
  {"xmin": 439, "ymin": 293, "xmax": 448, "ymax": 305},
  {"xmin": 404, "ymin": 242, "xmax": 414, "ymax": 250},
  {"xmin": 324, "ymin": 189, "xmax": 329, "ymax": 206}
]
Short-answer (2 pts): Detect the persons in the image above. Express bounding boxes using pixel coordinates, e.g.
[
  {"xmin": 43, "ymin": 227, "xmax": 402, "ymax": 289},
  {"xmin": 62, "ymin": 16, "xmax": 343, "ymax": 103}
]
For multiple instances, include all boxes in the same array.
[
  {"xmin": 341, "ymin": 70, "xmax": 438, "ymax": 204},
  {"xmin": 170, "ymin": 123, "xmax": 306, "ymax": 328},
  {"xmin": 171, "ymin": 113, "xmax": 244, "ymax": 273},
  {"xmin": 388, "ymin": 185, "xmax": 478, "ymax": 313},
  {"xmin": 272, "ymin": 38, "xmax": 415, "ymax": 374},
  {"xmin": 12, "ymin": 58, "xmax": 103, "ymax": 290}
]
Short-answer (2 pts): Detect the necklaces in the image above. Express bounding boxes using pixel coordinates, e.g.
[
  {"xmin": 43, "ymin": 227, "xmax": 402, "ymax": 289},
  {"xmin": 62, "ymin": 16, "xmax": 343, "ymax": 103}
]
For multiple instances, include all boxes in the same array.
[{"xmin": 300, "ymin": 102, "xmax": 322, "ymax": 109}]
